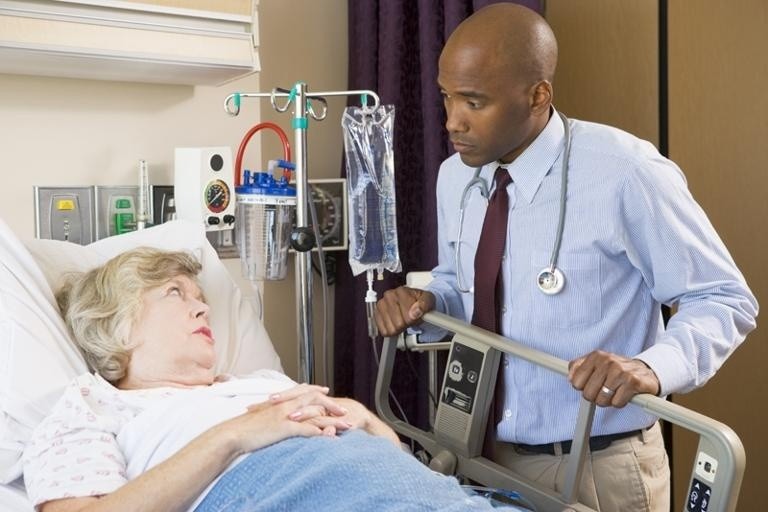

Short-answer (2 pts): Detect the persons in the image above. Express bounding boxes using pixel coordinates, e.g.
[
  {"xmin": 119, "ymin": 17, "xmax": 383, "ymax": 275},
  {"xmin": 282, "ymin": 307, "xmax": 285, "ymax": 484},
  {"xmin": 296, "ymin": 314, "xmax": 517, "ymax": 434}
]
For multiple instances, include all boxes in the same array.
[
  {"xmin": 22, "ymin": 247, "xmax": 401, "ymax": 512},
  {"xmin": 373, "ymin": 3, "xmax": 761, "ymax": 512}
]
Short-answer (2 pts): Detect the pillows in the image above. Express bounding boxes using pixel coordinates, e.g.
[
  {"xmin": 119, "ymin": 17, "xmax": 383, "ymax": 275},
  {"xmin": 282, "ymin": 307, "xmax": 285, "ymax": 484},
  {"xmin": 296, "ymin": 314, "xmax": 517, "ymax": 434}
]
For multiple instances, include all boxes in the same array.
[{"xmin": 2, "ymin": 205, "xmax": 284, "ymax": 486}]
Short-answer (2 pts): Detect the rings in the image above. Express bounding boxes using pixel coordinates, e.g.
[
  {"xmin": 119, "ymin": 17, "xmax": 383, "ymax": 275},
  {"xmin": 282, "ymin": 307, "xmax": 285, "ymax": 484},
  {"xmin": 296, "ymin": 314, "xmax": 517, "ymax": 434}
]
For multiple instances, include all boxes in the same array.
[{"xmin": 601, "ymin": 386, "xmax": 615, "ymax": 397}]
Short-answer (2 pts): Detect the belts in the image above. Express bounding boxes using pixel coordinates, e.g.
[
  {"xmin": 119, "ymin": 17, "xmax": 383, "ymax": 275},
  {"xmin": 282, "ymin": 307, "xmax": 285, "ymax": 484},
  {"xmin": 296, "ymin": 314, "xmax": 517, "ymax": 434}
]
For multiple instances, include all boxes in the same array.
[{"xmin": 515, "ymin": 422, "xmax": 654, "ymax": 455}]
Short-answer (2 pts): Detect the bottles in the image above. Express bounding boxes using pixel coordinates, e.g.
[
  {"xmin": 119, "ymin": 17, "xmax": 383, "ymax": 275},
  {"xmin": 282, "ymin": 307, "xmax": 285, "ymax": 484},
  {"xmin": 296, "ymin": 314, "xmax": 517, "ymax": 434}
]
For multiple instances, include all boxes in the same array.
[{"xmin": 235, "ymin": 171, "xmax": 296, "ymax": 281}]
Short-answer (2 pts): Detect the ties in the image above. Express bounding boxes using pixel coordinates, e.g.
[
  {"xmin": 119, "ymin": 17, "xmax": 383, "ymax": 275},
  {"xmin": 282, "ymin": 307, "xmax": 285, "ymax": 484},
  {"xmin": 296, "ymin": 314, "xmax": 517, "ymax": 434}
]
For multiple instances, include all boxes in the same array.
[{"xmin": 468, "ymin": 167, "xmax": 512, "ymax": 431}]
